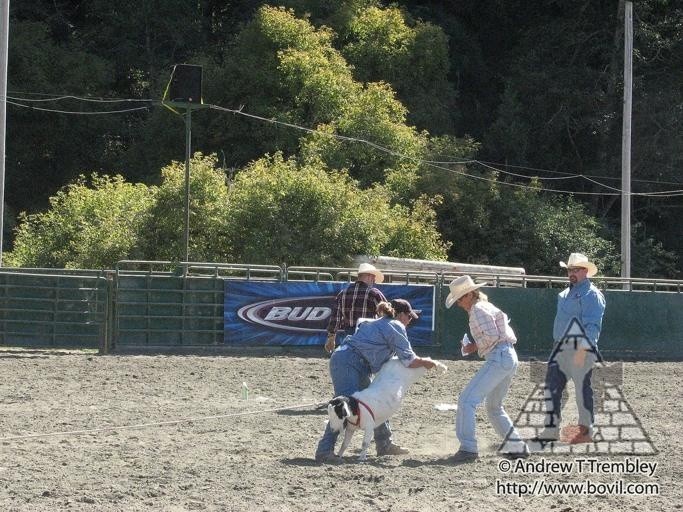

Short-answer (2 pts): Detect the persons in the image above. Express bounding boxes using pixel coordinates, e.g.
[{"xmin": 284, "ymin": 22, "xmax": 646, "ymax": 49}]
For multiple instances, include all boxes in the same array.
[
  {"xmin": 324, "ymin": 262, "xmax": 392, "ymax": 355},
  {"xmin": 538, "ymin": 253, "xmax": 607, "ymax": 442},
  {"xmin": 315, "ymin": 298, "xmax": 437, "ymax": 465},
  {"xmin": 445, "ymin": 273, "xmax": 530, "ymax": 461}
]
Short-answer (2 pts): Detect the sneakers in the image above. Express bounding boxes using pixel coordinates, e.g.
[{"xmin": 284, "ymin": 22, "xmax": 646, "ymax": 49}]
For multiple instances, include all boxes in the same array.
[
  {"xmin": 502, "ymin": 452, "xmax": 530, "ymax": 459},
  {"xmin": 531, "ymin": 427, "xmax": 560, "ymax": 441},
  {"xmin": 316, "ymin": 453, "xmax": 343, "ymax": 464},
  {"xmin": 378, "ymin": 445, "xmax": 409, "ymax": 455},
  {"xmin": 571, "ymin": 431, "xmax": 592, "ymax": 443},
  {"xmin": 448, "ymin": 451, "xmax": 478, "ymax": 461}
]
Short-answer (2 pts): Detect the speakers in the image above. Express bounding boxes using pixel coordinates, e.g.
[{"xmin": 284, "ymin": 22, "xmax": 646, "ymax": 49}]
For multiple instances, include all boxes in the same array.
[{"xmin": 169, "ymin": 64, "xmax": 203, "ymax": 104}]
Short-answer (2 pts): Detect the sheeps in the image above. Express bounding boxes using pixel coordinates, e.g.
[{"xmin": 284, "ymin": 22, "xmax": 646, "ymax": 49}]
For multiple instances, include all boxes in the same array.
[{"xmin": 327, "ymin": 355, "xmax": 447, "ymax": 461}]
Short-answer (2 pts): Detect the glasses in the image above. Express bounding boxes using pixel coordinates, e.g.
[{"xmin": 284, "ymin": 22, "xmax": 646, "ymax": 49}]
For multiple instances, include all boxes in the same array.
[{"xmin": 568, "ymin": 267, "xmax": 585, "ymax": 274}]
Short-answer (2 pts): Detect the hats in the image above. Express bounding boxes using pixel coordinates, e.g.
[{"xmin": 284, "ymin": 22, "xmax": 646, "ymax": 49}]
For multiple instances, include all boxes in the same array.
[
  {"xmin": 559, "ymin": 253, "xmax": 597, "ymax": 279},
  {"xmin": 391, "ymin": 299, "xmax": 420, "ymax": 320},
  {"xmin": 356, "ymin": 264, "xmax": 384, "ymax": 284},
  {"xmin": 445, "ymin": 275, "xmax": 487, "ymax": 309}
]
